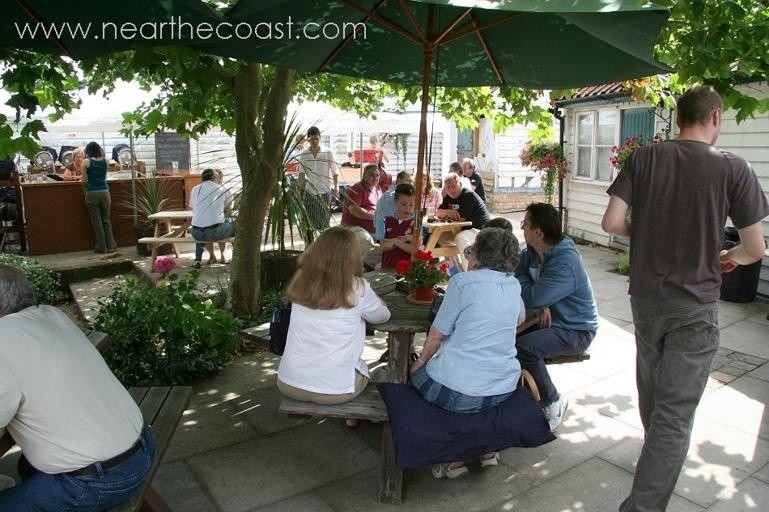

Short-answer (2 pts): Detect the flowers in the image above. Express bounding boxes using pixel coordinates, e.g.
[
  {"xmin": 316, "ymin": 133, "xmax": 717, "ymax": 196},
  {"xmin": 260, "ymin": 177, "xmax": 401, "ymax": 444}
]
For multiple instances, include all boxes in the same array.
[
  {"xmin": 397, "ymin": 249, "xmax": 449, "ymax": 289},
  {"xmin": 606, "ymin": 133, "xmax": 665, "ymax": 170},
  {"xmin": 518, "ymin": 138, "xmax": 569, "ymax": 206}
]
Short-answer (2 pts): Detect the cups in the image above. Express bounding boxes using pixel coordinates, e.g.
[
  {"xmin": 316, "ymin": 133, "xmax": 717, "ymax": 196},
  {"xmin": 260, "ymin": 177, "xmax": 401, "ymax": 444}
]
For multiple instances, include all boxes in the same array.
[
  {"xmin": 30, "ymin": 172, "xmax": 46, "ymax": 182},
  {"xmin": 172, "ymin": 162, "xmax": 178, "ymax": 169},
  {"xmin": 428, "ymin": 208, "xmax": 435, "ymax": 220}
]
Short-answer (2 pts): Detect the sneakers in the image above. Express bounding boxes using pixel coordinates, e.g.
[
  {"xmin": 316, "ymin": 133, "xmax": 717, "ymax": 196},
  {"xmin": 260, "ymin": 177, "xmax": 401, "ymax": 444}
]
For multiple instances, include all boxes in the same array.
[
  {"xmin": 192, "ymin": 254, "xmax": 229, "ymax": 268},
  {"xmin": 542, "ymin": 394, "xmax": 569, "ymax": 434},
  {"xmin": 479, "ymin": 451, "xmax": 500, "ymax": 468},
  {"xmin": 431, "ymin": 462, "xmax": 469, "ymax": 480}
]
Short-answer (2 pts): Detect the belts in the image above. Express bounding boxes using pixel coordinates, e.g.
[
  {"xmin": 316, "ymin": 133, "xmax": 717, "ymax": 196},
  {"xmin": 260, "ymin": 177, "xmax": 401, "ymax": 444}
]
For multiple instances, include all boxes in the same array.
[{"xmin": 67, "ymin": 424, "xmax": 152, "ymax": 475}]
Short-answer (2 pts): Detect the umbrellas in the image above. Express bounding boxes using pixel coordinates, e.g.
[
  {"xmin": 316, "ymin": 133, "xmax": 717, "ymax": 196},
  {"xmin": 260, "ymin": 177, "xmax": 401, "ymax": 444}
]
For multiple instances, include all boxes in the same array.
[
  {"xmin": 185, "ymin": 2, "xmax": 680, "ymax": 272},
  {"xmin": 0, "ymin": 0, "xmax": 261, "ymax": 62}
]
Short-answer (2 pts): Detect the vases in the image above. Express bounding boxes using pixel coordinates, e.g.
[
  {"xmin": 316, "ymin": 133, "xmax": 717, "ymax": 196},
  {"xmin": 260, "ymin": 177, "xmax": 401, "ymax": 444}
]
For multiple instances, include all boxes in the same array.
[{"xmin": 414, "ymin": 284, "xmax": 435, "ymax": 302}]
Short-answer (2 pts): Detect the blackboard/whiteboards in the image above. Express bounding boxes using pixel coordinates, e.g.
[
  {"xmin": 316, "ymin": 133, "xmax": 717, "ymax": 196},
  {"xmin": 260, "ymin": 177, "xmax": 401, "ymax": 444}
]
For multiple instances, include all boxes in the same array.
[{"xmin": 155, "ymin": 132, "xmax": 190, "ymax": 174}]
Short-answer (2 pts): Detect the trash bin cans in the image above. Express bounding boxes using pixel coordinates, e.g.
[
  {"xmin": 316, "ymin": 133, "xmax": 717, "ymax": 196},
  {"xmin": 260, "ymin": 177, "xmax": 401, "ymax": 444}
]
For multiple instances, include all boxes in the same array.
[{"xmin": 719, "ymin": 226, "xmax": 761, "ymax": 302}]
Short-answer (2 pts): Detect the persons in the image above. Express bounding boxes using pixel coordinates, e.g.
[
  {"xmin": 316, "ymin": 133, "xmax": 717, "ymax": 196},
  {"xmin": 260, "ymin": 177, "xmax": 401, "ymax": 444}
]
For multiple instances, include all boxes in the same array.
[
  {"xmin": 276, "ymin": 126, "xmax": 600, "ymax": 480},
  {"xmin": 80, "ymin": 142, "xmax": 118, "ymax": 254},
  {"xmin": 0, "ymin": 266, "xmax": 157, "ymax": 512},
  {"xmin": 188, "ymin": 169, "xmax": 236, "ymax": 268},
  {"xmin": 205, "ymin": 169, "xmax": 226, "ymax": 264},
  {"xmin": 63, "ymin": 149, "xmax": 84, "ymax": 181},
  {"xmin": 602, "ymin": 85, "xmax": 769, "ymax": 511}
]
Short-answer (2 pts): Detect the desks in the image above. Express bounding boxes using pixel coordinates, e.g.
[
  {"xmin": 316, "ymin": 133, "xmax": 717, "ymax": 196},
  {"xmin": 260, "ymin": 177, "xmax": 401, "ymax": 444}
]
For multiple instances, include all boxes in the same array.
[
  {"xmin": 361, "ymin": 267, "xmax": 446, "ymax": 384},
  {"xmin": 0, "ymin": 326, "xmax": 113, "ymax": 463},
  {"xmin": 422, "ymin": 214, "xmax": 473, "ymax": 258},
  {"xmin": 147, "ymin": 210, "xmax": 240, "ymax": 273}
]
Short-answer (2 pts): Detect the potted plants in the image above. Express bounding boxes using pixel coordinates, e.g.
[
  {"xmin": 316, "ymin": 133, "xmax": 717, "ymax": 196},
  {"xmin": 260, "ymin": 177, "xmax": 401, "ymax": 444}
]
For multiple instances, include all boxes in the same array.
[
  {"xmin": 113, "ymin": 166, "xmax": 186, "ymax": 256},
  {"xmin": 190, "ymin": 108, "xmax": 359, "ymax": 293}
]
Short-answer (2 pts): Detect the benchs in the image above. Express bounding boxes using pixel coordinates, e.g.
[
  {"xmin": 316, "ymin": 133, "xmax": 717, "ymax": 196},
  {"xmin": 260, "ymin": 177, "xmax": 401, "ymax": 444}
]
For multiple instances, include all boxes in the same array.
[
  {"xmin": 241, "ymin": 318, "xmax": 271, "ymax": 350},
  {"xmin": 277, "ymin": 381, "xmax": 541, "ymax": 505},
  {"xmin": 543, "ymin": 352, "xmax": 592, "ymax": 366},
  {"xmin": 137, "ymin": 236, "xmax": 235, "ymax": 274},
  {"xmin": 102, "ymin": 384, "xmax": 195, "ymax": 511}
]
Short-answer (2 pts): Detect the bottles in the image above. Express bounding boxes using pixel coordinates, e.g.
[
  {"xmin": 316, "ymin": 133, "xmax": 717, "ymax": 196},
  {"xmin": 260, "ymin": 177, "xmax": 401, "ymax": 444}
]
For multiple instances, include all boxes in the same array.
[
  {"xmin": 429, "ymin": 293, "xmax": 443, "ymax": 318},
  {"xmin": 273, "ymin": 304, "xmax": 282, "ymax": 323}
]
